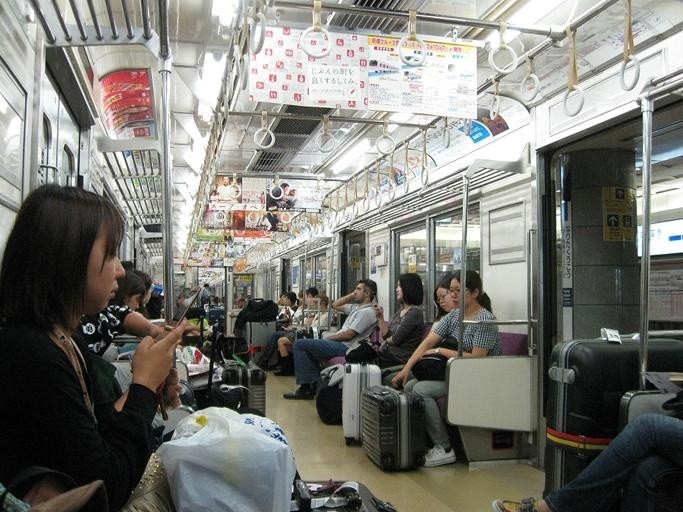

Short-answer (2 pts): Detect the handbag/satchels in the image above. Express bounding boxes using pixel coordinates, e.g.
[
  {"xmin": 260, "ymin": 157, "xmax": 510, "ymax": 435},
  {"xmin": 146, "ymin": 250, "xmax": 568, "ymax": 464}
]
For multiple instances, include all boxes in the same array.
[{"xmin": 317, "ymin": 368, "xmax": 343, "ymax": 425}]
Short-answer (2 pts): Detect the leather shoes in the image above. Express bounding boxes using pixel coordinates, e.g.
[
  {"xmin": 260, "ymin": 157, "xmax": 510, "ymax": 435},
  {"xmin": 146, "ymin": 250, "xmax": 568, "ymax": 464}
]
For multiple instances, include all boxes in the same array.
[
  {"xmin": 489, "ymin": 496, "xmax": 559, "ymax": 512},
  {"xmin": 272, "ymin": 359, "xmax": 292, "ymax": 376},
  {"xmin": 282, "ymin": 387, "xmax": 316, "ymax": 401}
]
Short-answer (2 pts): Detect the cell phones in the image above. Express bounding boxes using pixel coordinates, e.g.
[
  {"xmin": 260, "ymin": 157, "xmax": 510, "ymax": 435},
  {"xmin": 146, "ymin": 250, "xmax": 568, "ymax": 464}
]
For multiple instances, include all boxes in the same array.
[
  {"xmin": 372, "ymin": 291, "xmax": 378, "ymax": 303},
  {"xmin": 151, "ymin": 288, "xmax": 202, "ymax": 343}
]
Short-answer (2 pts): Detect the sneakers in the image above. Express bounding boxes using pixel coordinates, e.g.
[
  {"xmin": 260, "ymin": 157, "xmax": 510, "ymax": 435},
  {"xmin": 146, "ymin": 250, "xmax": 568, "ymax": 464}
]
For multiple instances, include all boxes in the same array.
[{"xmin": 420, "ymin": 444, "xmax": 457, "ymax": 469}]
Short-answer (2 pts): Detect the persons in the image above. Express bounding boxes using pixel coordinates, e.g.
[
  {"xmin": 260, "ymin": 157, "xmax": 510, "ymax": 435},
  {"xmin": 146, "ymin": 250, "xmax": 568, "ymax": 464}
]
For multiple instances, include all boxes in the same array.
[
  {"xmin": 216, "ymin": 176, "xmax": 236, "ymax": 200},
  {"xmin": 371, "ymin": 272, "xmax": 425, "ymax": 367},
  {"xmin": 283, "ymin": 279, "xmax": 381, "ymax": 400},
  {"xmin": 283, "ymin": 292, "xmax": 299, "ymax": 312},
  {"xmin": 391, "ymin": 270, "xmax": 503, "ymax": 468},
  {"xmin": 131, "ymin": 271, "xmax": 153, "ymax": 305},
  {"xmin": 265, "ymin": 183, "xmax": 289, "ymax": 209},
  {"xmin": 73, "ymin": 270, "xmax": 165, "ymax": 358},
  {"xmin": 106, "ymin": 103, "xmax": 151, "ymax": 127},
  {"xmin": 274, "ymin": 293, "xmax": 331, "ymax": 376},
  {"xmin": 266, "ymin": 287, "xmax": 319, "ymax": 371},
  {"xmin": 274, "ymin": 292, "xmax": 286, "ymax": 313},
  {"xmin": 494, "ymin": 411, "xmax": 683, "ymax": 511},
  {"xmin": 191, "ymin": 282, "xmax": 250, "ymax": 325},
  {"xmin": 376, "ymin": 271, "xmax": 456, "ymax": 388},
  {"xmin": 284, "ymin": 188, "xmax": 297, "ymax": 209},
  {"xmin": 260, "ymin": 209, "xmax": 282, "ymax": 232},
  {"xmin": 144, "ymin": 294, "xmax": 164, "ymax": 321},
  {"xmin": 0, "ymin": 183, "xmax": 182, "ymax": 511}
]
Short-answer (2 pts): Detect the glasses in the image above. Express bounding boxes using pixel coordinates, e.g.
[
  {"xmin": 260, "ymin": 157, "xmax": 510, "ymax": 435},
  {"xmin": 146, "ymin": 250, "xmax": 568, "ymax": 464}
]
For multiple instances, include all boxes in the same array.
[
  {"xmin": 446, "ymin": 286, "xmax": 471, "ymax": 297},
  {"xmin": 432, "ymin": 293, "xmax": 444, "ymax": 304}
]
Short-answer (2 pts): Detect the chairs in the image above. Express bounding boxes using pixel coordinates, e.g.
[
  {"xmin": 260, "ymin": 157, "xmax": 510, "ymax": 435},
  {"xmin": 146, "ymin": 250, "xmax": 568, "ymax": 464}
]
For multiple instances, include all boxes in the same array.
[{"xmin": 326, "ymin": 321, "xmax": 537, "ymax": 471}]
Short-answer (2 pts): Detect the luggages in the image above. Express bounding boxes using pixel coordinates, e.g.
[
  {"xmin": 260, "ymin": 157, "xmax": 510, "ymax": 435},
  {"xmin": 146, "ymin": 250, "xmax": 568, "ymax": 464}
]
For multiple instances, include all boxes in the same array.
[
  {"xmin": 537, "ymin": 328, "xmax": 683, "ymax": 504},
  {"xmin": 358, "ymin": 385, "xmax": 427, "ymax": 472},
  {"xmin": 221, "ymin": 364, "xmax": 268, "ymax": 418},
  {"xmin": 340, "ymin": 360, "xmax": 384, "ymax": 448},
  {"xmin": 242, "ymin": 296, "xmax": 280, "ymax": 368},
  {"xmin": 286, "ymin": 469, "xmax": 401, "ymax": 512}
]
